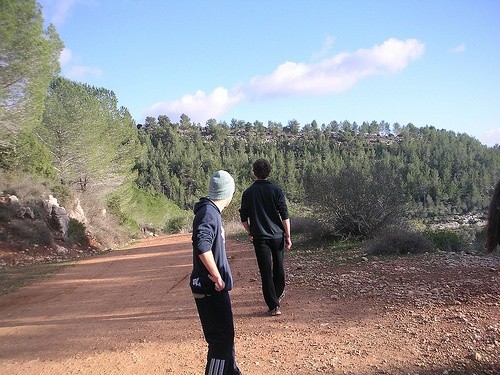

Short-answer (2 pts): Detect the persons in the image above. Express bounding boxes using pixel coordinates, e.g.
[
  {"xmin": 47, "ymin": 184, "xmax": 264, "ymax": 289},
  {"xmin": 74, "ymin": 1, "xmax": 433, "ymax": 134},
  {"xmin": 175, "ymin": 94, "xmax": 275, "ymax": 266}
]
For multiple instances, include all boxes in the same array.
[
  {"xmin": 237, "ymin": 159, "xmax": 294, "ymax": 315},
  {"xmin": 188, "ymin": 169, "xmax": 243, "ymax": 375}
]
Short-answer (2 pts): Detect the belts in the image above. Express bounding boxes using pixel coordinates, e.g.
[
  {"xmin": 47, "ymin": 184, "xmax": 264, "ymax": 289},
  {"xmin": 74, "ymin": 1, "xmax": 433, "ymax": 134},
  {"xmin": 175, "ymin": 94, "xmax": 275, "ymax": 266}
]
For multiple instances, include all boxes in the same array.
[{"xmin": 192, "ymin": 292, "xmax": 211, "ymax": 299}]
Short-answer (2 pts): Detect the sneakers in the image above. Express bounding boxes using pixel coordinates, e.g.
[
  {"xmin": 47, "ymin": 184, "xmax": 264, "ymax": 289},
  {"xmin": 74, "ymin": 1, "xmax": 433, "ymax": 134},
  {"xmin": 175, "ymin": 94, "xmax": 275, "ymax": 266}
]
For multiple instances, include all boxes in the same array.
[
  {"xmin": 266, "ymin": 306, "xmax": 281, "ymax": 315},
  {"xmin": 278, "ymin": 289, "xmax": 285, "ymax": 302}
]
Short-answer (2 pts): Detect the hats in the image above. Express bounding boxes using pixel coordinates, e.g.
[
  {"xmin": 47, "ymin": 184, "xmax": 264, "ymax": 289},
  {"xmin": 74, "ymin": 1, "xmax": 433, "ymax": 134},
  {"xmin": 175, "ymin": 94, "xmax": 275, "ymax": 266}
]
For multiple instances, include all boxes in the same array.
[{"xmin": 209, "ymin": 170, "xmax": 235, "ymax": 199}]
